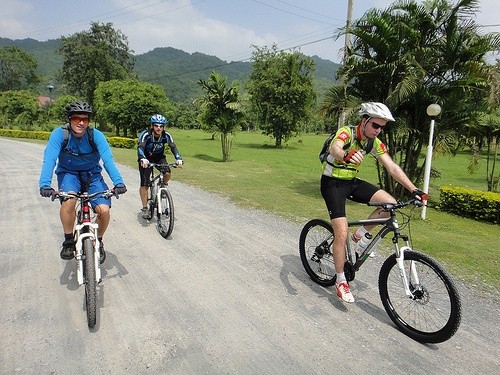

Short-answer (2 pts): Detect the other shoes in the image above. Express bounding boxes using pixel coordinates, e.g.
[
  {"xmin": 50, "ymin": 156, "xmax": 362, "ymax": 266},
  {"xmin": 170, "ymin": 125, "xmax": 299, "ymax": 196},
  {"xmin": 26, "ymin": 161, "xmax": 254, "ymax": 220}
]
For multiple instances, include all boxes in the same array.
[
  {"xmin": 162, "ymin": 183, "xmax": 168, "ymax": 199},
  {"xmin": 141, "ymin": 209, "xmax": 149, "ymax": 219}
]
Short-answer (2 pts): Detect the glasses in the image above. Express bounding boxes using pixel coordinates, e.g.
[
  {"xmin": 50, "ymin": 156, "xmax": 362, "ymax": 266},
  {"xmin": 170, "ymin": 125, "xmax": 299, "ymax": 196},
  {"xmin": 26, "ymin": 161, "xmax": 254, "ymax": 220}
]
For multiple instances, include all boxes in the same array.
[
  {"xmin": 154, "ymin": 125, "xmax": 163, "ymax": 127},
  {"xmin": 368, "ymin": 121, "xmax": 386, "ymax": 129},
  {"xmin": 69, "ymin": 117, "xmax": 90, "ymax": 125}
]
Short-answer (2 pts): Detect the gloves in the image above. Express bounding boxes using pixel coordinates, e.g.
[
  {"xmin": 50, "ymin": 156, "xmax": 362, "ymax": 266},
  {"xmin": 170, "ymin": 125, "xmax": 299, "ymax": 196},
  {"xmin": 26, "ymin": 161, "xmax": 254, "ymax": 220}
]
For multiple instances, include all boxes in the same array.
[
  {"xmin": 175, "ymin": 159, "xmax": 183, "ymax": 166},
  {"xmin": 113, "ymin": 184, "xmax": 126, "ymax": 199},
  {"xmin": 141, "ymin": 158, "xmax": 149, "ymax": 168},
  {"xmin": 40, "ymin": 186, "xmax": 55, "ymax": 201}
]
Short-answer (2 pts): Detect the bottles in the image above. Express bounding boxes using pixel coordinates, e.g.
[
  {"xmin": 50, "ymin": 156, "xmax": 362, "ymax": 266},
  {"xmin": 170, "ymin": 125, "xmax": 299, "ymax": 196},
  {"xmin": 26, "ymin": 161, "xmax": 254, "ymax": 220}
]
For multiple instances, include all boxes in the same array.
[{"xmin": 354, "ymin": 232, "xmax": 372, "ymax": 254}]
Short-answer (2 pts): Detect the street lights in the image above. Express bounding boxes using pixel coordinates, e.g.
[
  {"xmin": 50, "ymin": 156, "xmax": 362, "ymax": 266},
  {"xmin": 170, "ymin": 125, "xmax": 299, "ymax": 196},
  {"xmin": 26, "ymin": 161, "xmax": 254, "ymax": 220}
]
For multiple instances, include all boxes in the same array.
[
  {"xmin": 420, "ymin": 104, "xmax": 441, "ymax": 220},
  {"xmin": 47, "ymin": 85, "xmax": 54, "ymax": 103}
]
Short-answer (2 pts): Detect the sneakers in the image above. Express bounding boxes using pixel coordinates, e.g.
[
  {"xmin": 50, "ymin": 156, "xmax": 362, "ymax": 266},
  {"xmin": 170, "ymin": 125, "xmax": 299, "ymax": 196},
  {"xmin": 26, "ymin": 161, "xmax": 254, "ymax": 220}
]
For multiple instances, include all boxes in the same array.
[
  {"xmin": 335, "ymin": 281, "xmax": 354, "ymax": 303},
  {"xmin": 351, "ymin": 229, "xmax": 374, "ymax": 258},
  {"xmin": 100, "ymin": 241, "xmax": 106, "ymax": 264},
  {"xmin": 60, "ymin": 240, "xmax": 76, "ymax": 260}
]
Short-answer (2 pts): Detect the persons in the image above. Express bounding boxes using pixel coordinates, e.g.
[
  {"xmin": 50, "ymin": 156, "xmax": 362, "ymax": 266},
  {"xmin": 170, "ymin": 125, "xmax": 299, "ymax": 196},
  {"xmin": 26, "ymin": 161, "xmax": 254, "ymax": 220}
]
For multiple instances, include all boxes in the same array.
[
  {"xmin": 136, "ymin": 114, "xmax": 182, "ymax": 219},
  {"xmin": 320, "ymin": 102, "xmax": 429, "ymax": 304},
  {"xmin": 38, "ymin": 100, "xmax": 126, "ymax": 262}
]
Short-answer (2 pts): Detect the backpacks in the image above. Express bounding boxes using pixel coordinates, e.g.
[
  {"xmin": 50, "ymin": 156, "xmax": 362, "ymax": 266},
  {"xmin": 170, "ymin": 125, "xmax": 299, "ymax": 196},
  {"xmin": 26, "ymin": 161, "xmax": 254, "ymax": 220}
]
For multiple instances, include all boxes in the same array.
[{"xmin": 319, "ymin": 124, "xmax": 374, "ymax": 171}]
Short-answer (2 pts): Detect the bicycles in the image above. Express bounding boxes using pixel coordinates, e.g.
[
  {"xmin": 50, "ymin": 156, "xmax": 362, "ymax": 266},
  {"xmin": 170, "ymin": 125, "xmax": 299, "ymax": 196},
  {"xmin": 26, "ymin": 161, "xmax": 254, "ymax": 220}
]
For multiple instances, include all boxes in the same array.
[
  {"xmin": 299, "ymin": 195, "xmax": 462, "ymax": 344},
  {"xmin": 139, "ymin": 159, "xmax": 184, "ymax": 238},
  {"xmin": 52, "ymin": 184, "xmax": 115, "ymax": 329}
]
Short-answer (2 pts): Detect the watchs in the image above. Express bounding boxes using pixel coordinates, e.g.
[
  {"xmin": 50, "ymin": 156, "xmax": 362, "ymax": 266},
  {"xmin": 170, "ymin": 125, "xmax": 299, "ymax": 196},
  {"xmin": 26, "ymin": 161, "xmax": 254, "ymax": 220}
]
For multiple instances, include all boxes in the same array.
[{"xmin": 342, "ymin": 151, "xmax": 349, "ymax": 160}]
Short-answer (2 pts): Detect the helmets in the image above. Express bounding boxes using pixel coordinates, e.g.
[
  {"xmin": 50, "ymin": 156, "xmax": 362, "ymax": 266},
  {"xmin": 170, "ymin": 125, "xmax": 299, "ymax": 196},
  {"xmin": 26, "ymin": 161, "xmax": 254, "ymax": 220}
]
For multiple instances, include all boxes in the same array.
[
  {"xmin": 359, "ymin": 102, "xmax": 395, "ymax": 122},
  {"xmin": 151, "ymin": 114, "xmax": 166, "ymax": 124},
  {"xmin": 67, "ymin": 101, "xmax": 92, "ymax": 113}
]
